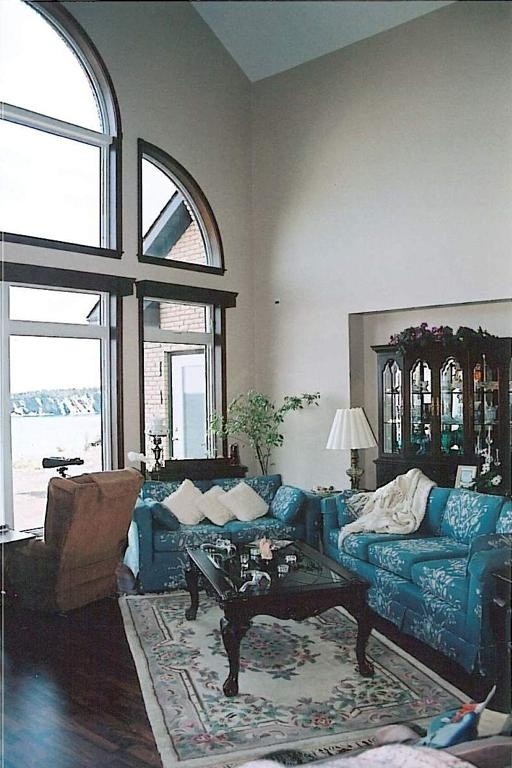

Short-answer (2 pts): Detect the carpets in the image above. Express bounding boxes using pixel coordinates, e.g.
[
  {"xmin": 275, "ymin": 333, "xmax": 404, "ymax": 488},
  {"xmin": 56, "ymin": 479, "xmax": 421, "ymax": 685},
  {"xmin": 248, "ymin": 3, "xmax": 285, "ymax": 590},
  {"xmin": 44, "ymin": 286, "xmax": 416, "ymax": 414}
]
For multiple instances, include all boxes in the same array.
[{"xmin": 114, "ymin": 591, "xmax": 511, "ymax": 767}]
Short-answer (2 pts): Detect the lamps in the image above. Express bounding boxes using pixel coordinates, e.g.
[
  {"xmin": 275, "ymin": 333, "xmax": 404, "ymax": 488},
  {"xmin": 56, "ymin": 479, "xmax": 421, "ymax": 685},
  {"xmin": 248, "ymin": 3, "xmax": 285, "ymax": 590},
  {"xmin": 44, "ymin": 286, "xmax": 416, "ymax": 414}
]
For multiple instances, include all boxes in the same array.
[
  {"xmin": 326, "ymin": 408, "xmax": 376, "ymax": 497},
  {"xmin": 147, "ymin": 419, "xmax": 168, "ymax": 473}
]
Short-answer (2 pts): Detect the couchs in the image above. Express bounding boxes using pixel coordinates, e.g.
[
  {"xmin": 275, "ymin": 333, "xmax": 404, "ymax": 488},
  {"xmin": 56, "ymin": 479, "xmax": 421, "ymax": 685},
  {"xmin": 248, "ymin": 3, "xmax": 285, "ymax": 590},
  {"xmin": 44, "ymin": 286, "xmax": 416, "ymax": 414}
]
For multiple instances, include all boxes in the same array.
[
  {"xmin": 322, "ymin": 485, "xmax": 512, "ymax": 704},
  {"xmin": 135, "ymin": 474, "xmax": 320, "ymax": 593}
]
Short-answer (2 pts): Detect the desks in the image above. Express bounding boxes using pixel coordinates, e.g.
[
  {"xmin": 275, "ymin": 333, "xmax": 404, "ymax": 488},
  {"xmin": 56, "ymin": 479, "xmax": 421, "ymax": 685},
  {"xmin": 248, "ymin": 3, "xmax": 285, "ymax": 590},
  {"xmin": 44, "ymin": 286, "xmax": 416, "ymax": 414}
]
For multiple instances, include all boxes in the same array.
[
  {"xmin": 0, "ymin": 528, "xmax": 37, "ymax": 545},
  {"xmin": 490, "ymin": 566, "xmax": 512, "ymax": 713}
]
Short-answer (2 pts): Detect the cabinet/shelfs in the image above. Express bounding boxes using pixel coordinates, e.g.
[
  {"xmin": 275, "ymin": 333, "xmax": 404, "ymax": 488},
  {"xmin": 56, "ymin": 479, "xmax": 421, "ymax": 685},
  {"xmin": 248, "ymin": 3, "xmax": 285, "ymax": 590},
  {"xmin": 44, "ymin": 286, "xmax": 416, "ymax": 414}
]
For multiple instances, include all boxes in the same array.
[{"xmin": 370, "ymin": 337, "xmax": 512, "ymax": 498}]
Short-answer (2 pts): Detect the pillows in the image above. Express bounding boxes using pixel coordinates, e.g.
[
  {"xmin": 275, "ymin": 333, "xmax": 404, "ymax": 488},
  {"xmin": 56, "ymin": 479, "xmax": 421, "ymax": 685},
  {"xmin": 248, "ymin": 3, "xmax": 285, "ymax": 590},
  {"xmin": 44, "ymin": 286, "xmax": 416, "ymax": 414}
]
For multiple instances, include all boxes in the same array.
[
  {"xmin": 162, "ymin": 477, "xmax": 206, "ymax": 525},
  {"xmin": 196, "ymin": 485, "xmax": 236, "ymax": 526},
  {"xmin": 269, "ymin": 485, "xmax": 306, "ymax": 524},
  {"xmin": 218, "ymin": 482, "xmax": 269, "ymax": 522},
  {"xmin": 145, "ymin": 497, "xmax": 180, "ymax": 531}
]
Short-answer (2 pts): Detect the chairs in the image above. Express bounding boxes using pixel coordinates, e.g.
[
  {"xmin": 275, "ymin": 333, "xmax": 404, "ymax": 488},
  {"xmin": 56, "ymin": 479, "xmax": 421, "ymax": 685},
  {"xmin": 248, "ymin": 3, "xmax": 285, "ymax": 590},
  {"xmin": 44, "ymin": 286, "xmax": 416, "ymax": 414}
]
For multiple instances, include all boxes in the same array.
[{"xmin": 0, "ymin": 468, "xmax": 142, "ymax": 614}]
